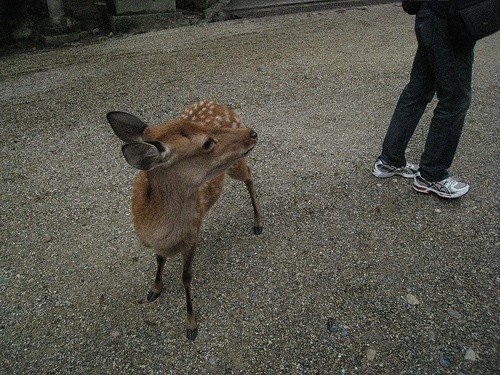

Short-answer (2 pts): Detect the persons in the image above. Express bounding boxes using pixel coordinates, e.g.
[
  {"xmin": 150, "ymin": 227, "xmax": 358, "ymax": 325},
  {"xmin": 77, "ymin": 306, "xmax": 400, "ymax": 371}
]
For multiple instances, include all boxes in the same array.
[{"xmin": 373, "ymin": 1, "xmax": 499, "ymax": 199}]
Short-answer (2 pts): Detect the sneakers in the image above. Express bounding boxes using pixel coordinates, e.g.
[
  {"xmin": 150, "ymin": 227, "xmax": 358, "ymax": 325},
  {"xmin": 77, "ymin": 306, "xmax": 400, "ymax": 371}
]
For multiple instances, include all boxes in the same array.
[
  {"xmin": 413, "ymin": 168, "xmax": 470, "ymax": 199},
  {"xmin": 371, "ymin": 157, "xmax": 421, "ymax": 178}
]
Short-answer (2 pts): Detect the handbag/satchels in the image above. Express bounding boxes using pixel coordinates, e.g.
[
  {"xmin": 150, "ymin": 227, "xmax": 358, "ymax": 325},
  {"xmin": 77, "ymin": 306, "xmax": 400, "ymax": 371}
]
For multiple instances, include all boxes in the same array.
[{"xmin": 454, "ymin": 1, "xmax": 499, "ymax": 41}]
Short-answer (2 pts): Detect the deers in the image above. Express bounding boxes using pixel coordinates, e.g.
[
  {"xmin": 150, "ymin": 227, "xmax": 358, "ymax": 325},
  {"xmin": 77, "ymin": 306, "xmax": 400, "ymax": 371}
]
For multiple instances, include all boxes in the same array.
[{"xmin": 106, "ymin": 100, "xmax": 264, "ymax": 341}]
